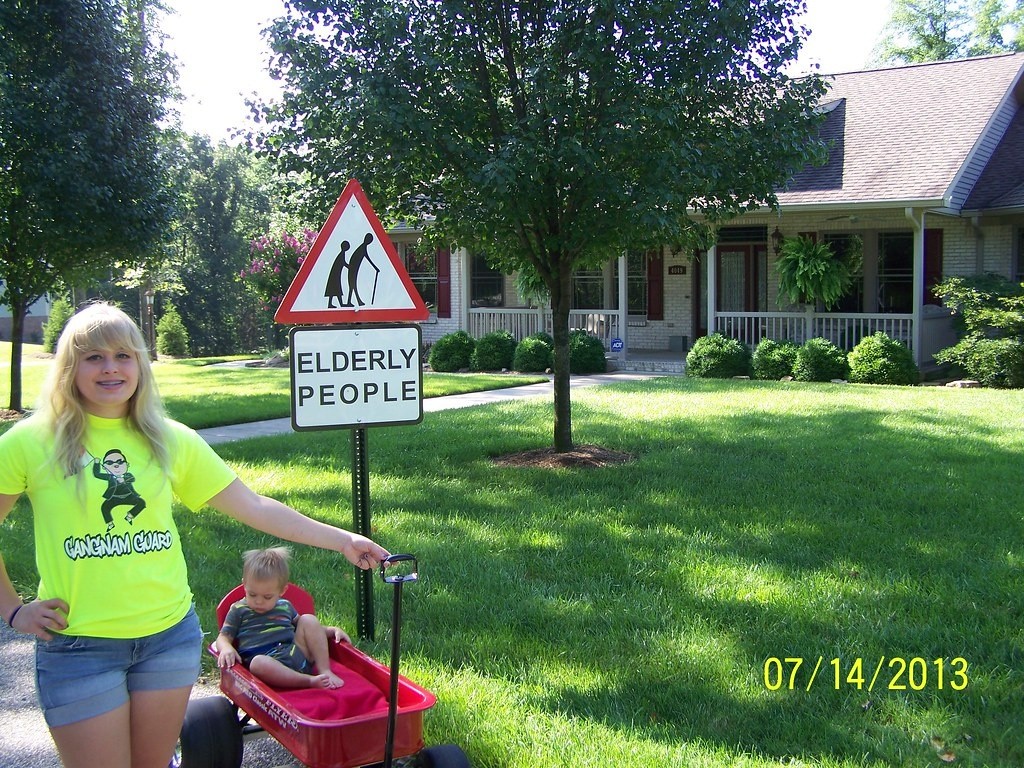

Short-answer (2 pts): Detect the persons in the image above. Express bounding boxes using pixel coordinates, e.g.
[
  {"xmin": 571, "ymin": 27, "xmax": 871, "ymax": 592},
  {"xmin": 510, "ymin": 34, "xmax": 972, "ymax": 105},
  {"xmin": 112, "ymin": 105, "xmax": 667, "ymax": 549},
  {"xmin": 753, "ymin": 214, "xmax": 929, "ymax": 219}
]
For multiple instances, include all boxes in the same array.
[
  {"xmin": 0, "ymin": 304, "xmax": 399, "ymax": 768},
  {"xmin": 216, "ymin": 546, "xmax": 351, "ymax": 690}
]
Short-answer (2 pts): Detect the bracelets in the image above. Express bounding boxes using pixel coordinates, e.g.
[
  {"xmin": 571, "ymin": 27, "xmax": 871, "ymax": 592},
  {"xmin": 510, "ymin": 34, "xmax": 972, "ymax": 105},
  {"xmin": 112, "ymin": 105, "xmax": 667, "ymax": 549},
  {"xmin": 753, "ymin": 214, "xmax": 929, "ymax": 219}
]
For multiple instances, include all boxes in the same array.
[{"xmin": 9, "ymin": 606, "xmax": 22, "ymax": 628}]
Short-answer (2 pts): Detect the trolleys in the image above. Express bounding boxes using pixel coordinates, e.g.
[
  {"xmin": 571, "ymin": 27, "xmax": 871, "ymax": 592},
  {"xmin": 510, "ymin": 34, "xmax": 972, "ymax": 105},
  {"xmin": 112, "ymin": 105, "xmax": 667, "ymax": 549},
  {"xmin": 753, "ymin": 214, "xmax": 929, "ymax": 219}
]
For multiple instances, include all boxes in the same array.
[{"xmin": 168, "ymin": 554, "xmax": 470, "ymax": 768}]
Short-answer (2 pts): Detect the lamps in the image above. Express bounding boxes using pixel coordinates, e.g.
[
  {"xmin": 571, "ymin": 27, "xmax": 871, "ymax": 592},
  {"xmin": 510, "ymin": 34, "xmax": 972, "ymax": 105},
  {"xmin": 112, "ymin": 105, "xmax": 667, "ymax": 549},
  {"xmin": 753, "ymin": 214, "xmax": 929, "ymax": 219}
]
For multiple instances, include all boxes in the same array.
[{"xmin": 771, "ymin": 226, "xmax": 783, "ymax": 255}]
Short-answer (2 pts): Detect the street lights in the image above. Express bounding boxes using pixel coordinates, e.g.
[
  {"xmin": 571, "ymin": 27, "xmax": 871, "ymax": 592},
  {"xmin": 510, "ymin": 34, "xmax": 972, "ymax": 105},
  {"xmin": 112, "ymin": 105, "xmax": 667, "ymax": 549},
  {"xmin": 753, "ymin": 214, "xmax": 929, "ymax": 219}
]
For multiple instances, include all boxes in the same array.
[{"xmin": 143, "ymin": 288, "xmax": 156, "ymax": 362}]
